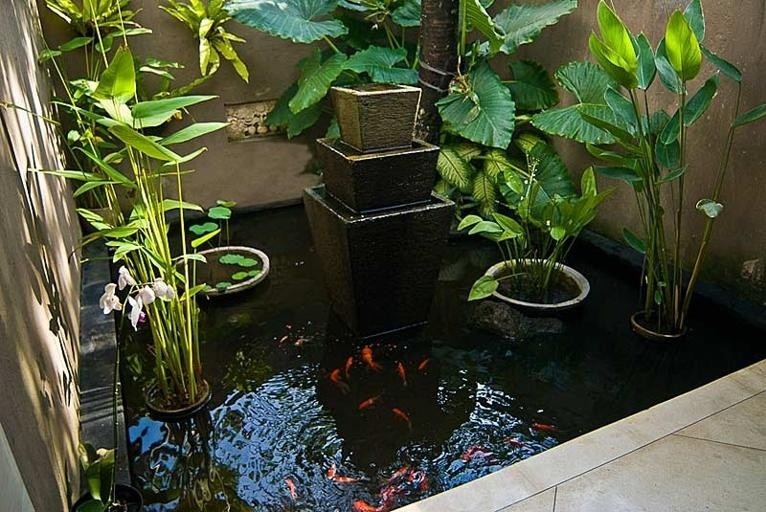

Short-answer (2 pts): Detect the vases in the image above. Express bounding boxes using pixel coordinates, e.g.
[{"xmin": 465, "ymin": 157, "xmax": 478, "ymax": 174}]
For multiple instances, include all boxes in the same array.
[{"xmin": 71, "ymin": 482, "xmax": 144, "ymax": 512}]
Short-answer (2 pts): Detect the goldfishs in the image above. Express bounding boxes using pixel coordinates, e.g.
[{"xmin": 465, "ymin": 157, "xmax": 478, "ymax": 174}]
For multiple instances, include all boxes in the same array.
[{"xmin": 326, "ymin": 460, "xmax": 428, "ymax": 512}]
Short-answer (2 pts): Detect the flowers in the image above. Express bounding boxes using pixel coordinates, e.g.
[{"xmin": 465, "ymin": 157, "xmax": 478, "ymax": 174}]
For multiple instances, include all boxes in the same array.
[{"xmin": 98, "ymin": 265, "xmax": 176, "ymax": 508}]
